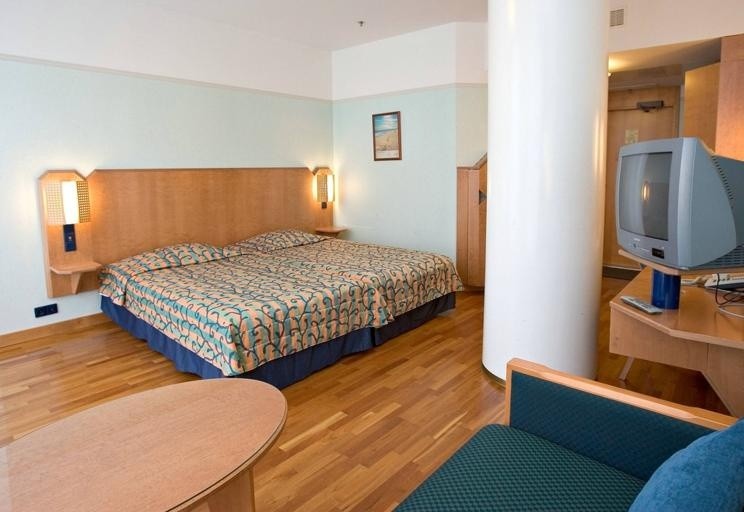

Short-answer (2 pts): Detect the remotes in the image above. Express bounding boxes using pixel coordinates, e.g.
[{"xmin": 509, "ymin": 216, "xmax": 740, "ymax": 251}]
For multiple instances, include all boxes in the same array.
[{"xmin": 620, "ymin": 294, "xmax": 663, "ymax": 316}]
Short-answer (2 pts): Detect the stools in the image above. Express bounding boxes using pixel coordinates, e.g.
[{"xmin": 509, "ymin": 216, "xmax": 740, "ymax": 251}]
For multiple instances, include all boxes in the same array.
[{"xmin": 387, "ymin": 354, "xmax": 744, "ymax": 512}]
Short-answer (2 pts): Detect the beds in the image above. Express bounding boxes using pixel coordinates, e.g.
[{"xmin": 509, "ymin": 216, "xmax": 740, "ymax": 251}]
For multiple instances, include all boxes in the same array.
[{"xmin": 92, "ymin": 223, "xmax": 466, "ymax": 390}]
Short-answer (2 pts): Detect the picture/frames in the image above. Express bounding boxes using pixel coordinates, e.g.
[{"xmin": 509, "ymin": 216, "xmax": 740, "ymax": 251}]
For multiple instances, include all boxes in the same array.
[{"xmin": 370, "ymin": 110, "xmax": 402, "ymax": 162}]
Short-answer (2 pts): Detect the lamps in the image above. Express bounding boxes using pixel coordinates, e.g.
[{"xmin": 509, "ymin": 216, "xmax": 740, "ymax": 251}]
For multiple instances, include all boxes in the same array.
[
  {"xmin": 40, "ymin": 176, "xmax": 92, "ymax": 254},
  {"xmin": 315, "ymin": 173, "xmax": 335, "ymax": 211}
]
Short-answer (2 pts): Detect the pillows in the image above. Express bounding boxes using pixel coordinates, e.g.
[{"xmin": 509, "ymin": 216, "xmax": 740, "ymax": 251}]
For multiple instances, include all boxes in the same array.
[
  {"xmin": 622, "ymin": 411, "xmax": 743, "ymax": 512},
  {"xmin": 94, "ymin": 240, "xmax": 242, "ymax": 308},
  {"xmin": 218, "ymin": 228, "xmax": 336, "ymax": 256}
]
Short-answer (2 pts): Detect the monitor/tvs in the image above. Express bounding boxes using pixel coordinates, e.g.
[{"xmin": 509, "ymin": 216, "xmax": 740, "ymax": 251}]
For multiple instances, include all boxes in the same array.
[{"xmin": 615, "ymin": 136, "xmax": 744, "ymax": 272}]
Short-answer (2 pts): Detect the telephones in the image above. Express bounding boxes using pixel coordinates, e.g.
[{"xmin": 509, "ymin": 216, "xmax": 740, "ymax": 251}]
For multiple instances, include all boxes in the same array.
[{"xmin": 704, "ymin": 272, "xmax": 744, "ymax": 289}]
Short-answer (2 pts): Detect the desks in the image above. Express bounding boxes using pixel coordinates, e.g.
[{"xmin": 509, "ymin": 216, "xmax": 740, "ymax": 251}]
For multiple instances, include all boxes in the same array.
[
  {"xmin": 47, "ymin": 259, "xmax": 103, "ymax": 297},
  {"xmin": 0, "ymin": 377, "xmax": 289, "ymax": 510},
  {"xmin": 605, "ymin": 261, "xmax": 744, "ymax": 427},
  {"xmin": 313, "ymin": 224, "xmax": 349, "ymax": 240}
]
